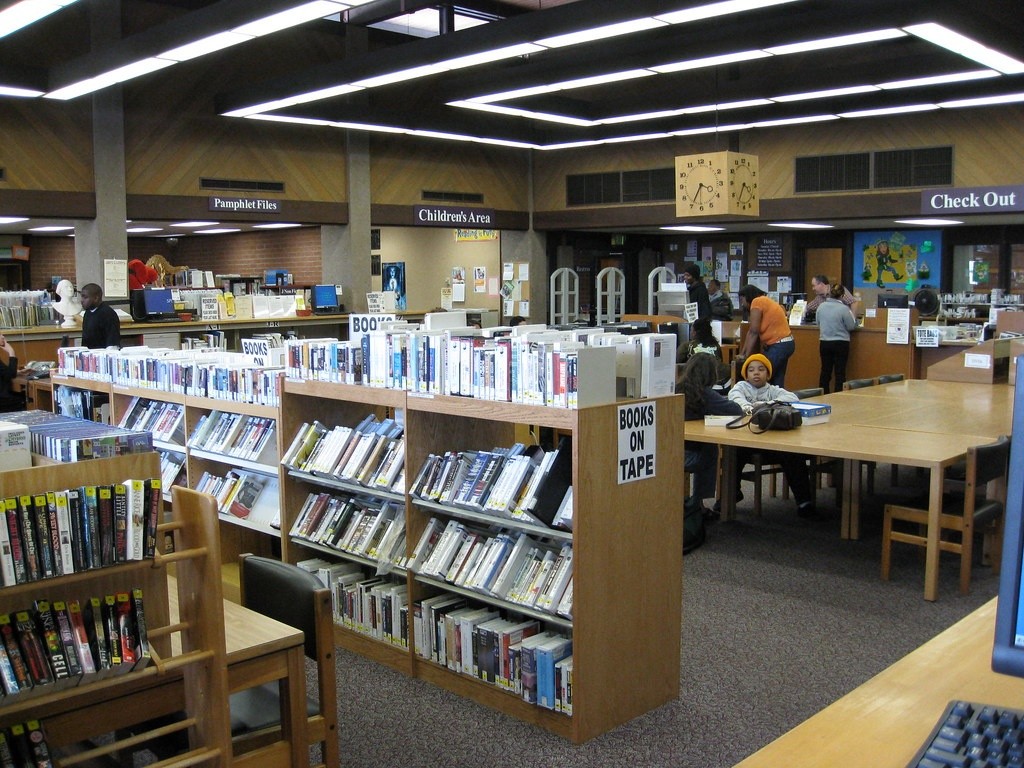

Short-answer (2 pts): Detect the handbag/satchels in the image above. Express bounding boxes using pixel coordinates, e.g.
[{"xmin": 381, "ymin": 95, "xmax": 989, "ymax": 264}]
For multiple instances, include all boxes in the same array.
[{"xmin": 725, "ymin": 402, "xmax": 802, "ymax": 435}]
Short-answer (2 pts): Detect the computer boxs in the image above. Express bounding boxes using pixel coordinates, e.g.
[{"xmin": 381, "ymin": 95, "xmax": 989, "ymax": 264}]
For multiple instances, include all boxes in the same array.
[
  {"xmin": 678, "ymin": 323, "xmax": 693, "ymax": 345},
  {"xmin": 130, "ymin": 289, "xmax": 146, "ymax": 321},
  {"xmin": 623, "ymin": 321, "xmax": 652, "ymax": 333}
]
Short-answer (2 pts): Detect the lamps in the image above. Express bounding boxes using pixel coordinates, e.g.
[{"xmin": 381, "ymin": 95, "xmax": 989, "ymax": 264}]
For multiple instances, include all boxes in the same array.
[{"xmin": 0, "ymin": 0, "xmax": 1024, "ymax": 148}]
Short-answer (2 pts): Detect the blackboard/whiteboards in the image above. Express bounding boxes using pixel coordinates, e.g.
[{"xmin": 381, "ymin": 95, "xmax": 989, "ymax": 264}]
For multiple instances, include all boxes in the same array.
[{"xmin": 749, "ymin": 234, "xmax": 791, "ymax": 272}]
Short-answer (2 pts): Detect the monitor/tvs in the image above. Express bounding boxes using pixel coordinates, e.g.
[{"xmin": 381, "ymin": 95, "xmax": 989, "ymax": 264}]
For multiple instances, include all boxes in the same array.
[
  {"xmin": 312, "ymin": 285, "xmax": 338, "ymax": 312},
  {"xmin": 878, "ymin": 294, "xmax": 908, "ymax": 308},
  {"xmin": 143, "ymin": 288, "xmax": 175, "ymax": 318},
  {"xmin": 617, "ymin": 323, "xmax": 680, "ymax": 348},
  {"xmin": 989, "ymin": 356, "xmax": 1024, "ymax": 678}
]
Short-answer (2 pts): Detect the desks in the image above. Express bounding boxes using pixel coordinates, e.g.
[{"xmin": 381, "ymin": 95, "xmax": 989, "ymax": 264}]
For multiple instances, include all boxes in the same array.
[
  {"xmin": 164, "ymin": 578, "xmax": 310, "ymax": 765},
  {"xmin": 801, "ymin": 395, "xmax": 1011, "ymax": 441},
  {"xmin": 864, "ymin": 380, "xmax": 1015, "ymax": 410},
  {"xmin": 686, "ymin": 421, "xmax": 999, "ymax": 603},
  {"xmin": 728, "ymin": 596, "xmax": 1024, "ymax": 768}
]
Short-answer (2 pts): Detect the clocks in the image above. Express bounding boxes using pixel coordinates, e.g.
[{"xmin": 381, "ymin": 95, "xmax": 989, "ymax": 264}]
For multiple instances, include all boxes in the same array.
[
  {"xmin": 728, "ymin": 153, "xmax": 759, "ymax": 215},
  {"xmin": 672, "ymin": 151, "xmax": 728, "ymax": 216}
]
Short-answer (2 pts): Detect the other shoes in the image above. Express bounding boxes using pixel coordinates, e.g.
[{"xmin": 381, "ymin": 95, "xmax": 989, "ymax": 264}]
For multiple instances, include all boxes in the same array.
[
  {"xmin": 714, "ymin": 488, "xmax": 744, "ymax": 511},
  {"xmin": 797, "ymin": 503, "xmax": 822, "ymax": 521}
]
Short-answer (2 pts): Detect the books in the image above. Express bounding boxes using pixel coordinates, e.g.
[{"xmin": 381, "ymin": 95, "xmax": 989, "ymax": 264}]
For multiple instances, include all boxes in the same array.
[
  {"xmin": 0, "ymin": 291, "xmax": 53, "ymax": 326},
  {"xmin": 0, "ymin": 410, "xmax": 161, "ymax": 768},
  {"xmin": 55, "ymin": 331, "xmax": 676, "ymax": 716}
]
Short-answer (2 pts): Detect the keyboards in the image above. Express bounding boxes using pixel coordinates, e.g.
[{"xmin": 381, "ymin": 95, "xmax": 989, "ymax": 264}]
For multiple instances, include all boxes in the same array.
[
  {"xmin": 147, "ymin": 317, "xmax": 184, "ymax": 322},
  {"xmin": 905, "ymin": 700, "xmax": 1023, "ymax": 768}
]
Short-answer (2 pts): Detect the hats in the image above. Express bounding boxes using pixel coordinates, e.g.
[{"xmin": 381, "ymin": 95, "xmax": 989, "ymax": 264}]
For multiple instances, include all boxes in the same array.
[
  {"xmin": 741, "ymin": 354, "xmax": 772, "ymax": 381},
  {"xmin": 685, "ymin": 264, "xmax": 700, "ymax": 280}
]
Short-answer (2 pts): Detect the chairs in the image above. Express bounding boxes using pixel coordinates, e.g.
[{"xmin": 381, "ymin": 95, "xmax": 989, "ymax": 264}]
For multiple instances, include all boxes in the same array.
[
  {"xmin": 684, "ymin": 376, "xmax": 1014, "ymax": 593},
  {"xmin": 232, "ymin": 549, "xmax": 347, "ymax": 766}
]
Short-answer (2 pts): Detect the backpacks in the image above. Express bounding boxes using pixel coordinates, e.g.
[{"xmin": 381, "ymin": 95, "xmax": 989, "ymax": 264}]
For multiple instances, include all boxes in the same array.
[{"xmin": 683, "ymin": 496, "xmax": 712, "ymax": 555}]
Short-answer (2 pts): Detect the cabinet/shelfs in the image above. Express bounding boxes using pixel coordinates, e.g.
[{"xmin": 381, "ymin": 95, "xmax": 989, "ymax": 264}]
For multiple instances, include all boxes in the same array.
[
  {"xmin": 936, "ymin": 289, "xmax": 1021, "ymax": 322},
  {"xmin": 0, "ymin": 480, "xmax": 236, "ymax": 766},
  {"xmin": 279, "ymin": 361, "xmax": 684, "ymax": 745},
  {"xmin": 38, "ymin": 358, "xmax": 277, "ymax": 572}
]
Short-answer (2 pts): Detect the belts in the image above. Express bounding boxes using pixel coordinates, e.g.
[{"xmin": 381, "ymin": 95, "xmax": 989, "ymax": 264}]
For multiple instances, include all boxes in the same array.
[{"xmin": 777, "ymin": 334, "xmax": 794, "ymax": 344}]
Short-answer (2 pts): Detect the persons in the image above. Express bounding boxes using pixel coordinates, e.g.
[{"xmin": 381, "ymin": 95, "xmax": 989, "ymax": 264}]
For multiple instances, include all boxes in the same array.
[
  {"xmin": 735, "ymin": 285, "xmax": 795, "ymax": 387},
  {"xmin": 690, "ymin": 318, "xmax": 717, "ymax": 355},
  {"xmin": 52, "ymin": 280, "xmax": 83, "ymax": 316},
  {"xmin": 712, "ymin": 353, "xmax": 830, "ymax": 522},
  {"xmin": 806, "ymin": 275, "xmax": 859, "ymax": 394},
  {"xmin": 684, "ymin": 264, "xmax": 713, "ymax": 320},
  {"xmin": 510, "ymin": 316, "xmax": 527, "ymax": 327},
  {"xmin": 675, "ymin": 353, "xmax": 741, "ymax": 500},
  {"xmin": 0, "ymin": 334, "xmax": 27, "ymax": 411},
  {"xmin": 708, "ymin": 280, "xmax": 733, "ymax": 320},
  {"xmin": 81, "ymin": 283, "xmax": 120, "ymax": 348}
]
